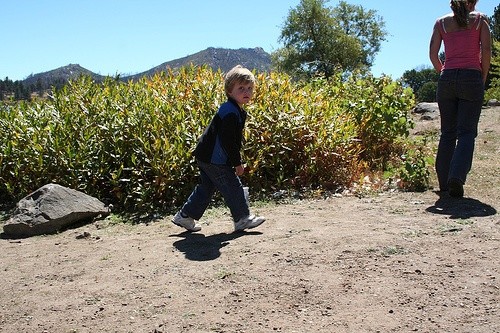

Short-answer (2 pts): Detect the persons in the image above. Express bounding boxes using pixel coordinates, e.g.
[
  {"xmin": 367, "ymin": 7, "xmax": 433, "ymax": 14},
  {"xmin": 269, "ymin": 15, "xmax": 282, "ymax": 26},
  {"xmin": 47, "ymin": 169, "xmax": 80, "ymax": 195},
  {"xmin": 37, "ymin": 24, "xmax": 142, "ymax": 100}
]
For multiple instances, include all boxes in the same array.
[
  {"xmin": 170, "ymin": 62, "xmax": 267, "ymax": 233},
  {"xmin": 429, "ymin": 1, "xmax": 491, "ymax": 198}
]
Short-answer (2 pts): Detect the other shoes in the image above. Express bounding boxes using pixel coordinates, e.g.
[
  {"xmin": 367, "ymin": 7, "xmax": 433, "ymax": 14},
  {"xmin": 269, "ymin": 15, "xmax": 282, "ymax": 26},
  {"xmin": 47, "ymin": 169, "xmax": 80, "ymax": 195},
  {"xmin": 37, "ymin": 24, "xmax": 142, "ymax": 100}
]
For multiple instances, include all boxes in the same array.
[
  {"xmin": 440, "ymin": 185, "xmax": 451, "ymax": 202},
  {"xmin": 449, "ymin": 186, "xmax": 464, "ymax": 201}
]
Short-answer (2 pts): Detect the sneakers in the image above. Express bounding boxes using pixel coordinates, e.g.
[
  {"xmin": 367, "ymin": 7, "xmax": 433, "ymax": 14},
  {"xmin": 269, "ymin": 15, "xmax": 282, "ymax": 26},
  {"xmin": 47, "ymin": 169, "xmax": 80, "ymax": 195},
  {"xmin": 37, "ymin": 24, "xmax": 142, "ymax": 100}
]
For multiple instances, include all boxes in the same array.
[
  {"xmin": 236, "ymin": 215, "xmax": 266, "ymax": 231},
  {"xmin": 171, "ymin": 211, "xmax": 201, "ymax": 232}
]
